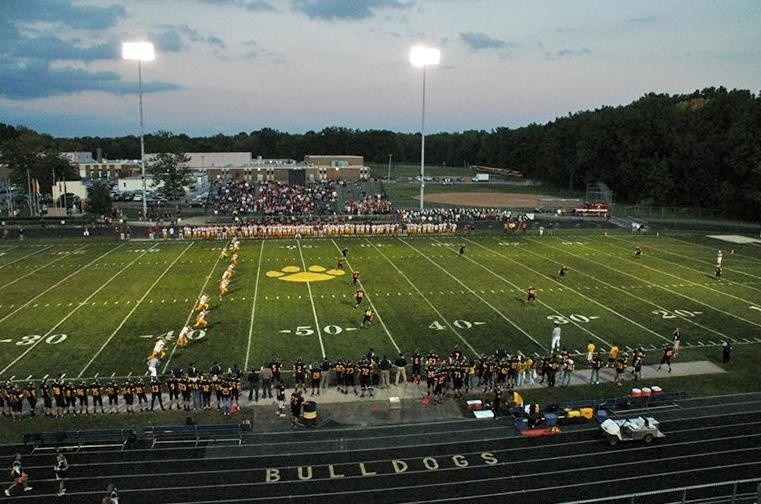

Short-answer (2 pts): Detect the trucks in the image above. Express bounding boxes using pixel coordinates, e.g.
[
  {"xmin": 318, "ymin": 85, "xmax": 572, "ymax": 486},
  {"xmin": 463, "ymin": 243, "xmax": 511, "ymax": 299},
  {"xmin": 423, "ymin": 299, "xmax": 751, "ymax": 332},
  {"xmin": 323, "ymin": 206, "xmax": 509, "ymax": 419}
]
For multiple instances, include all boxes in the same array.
[{"xmin": 472, "ymin": 173, "xmax": 490, "ymax": 182}]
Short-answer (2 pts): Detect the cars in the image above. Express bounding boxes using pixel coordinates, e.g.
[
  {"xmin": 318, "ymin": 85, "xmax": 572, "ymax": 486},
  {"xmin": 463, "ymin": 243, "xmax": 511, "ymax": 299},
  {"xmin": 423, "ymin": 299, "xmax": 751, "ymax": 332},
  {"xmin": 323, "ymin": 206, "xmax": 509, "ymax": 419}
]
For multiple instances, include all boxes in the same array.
[
  {"xmin": 187, "ymin": 192, "xmax": 209, "ymax": 208},
  {"xmin": 109, "ymin": 189, "xmax": 167, "ymax": 206},
  {"xmin": 407, "ymin": 175, "xmax": 465, "ymax": 182}
]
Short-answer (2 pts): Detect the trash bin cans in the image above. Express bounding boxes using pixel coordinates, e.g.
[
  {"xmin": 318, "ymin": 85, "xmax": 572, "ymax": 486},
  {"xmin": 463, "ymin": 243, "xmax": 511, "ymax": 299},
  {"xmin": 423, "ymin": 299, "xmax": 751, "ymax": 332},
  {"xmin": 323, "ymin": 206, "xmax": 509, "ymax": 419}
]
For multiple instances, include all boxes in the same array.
[{"xmin": 303, "ymin": 401, "xmax": 318, "ymax": 425}]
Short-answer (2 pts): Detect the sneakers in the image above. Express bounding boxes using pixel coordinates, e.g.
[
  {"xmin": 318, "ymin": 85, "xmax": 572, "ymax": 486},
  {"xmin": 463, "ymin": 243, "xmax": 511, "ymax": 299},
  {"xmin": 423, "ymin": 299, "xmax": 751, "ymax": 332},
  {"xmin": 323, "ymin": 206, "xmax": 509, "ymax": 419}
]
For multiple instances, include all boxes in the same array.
[
  {"xmin": 247, "ymin": 380, "xmax": 421, "ymax": 402},
  {"xmin": 24, "ymin": 486, "xmax": 33, "ymax": 491},
  {"xmin": 4, "ymin": 489, "xmax": 11, "ymax": 496},
  {"xmin": 419, "ymin": 365, "xmax": 570, "ymax": 404},
  {"xmin": 59, "ymin": 488, "xmax": 67, "ymax": 492},
  {"xmin": 2, "ymin": 402, "xmax": 239, "ymax": 422},
  {"xmin": 58, "ymin": 491, "xmax": 65, "ymax": 496}
]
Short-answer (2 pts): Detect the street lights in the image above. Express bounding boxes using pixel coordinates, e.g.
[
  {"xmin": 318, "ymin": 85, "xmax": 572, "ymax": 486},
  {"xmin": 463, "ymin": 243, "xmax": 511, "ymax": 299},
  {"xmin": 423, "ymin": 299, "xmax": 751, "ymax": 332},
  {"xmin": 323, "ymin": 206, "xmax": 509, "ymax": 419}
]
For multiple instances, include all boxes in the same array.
[
  {"xmin": 122, "ymin": 41, "xmax": 156, "ymax": 219},
  {"xmin": 387, "ymin": 153, "xmax": 393, "ymax": 182},
  {"xmin": 410, "ymin": 45, "xmax": 440, "ymax": 211}
]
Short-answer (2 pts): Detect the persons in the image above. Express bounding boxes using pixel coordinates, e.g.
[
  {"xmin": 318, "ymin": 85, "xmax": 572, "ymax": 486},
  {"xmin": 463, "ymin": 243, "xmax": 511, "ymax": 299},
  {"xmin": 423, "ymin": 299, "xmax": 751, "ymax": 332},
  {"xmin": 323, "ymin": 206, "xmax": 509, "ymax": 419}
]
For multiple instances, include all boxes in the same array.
[
  {"xmin": 556, "ymin": 265, "xmax": 569, "ymax": 282},
  {"xmin": 527, "ymin": 285, "xmax": 536, "ymax": 305},
  {"xmin": 351, "ymin": 269, "xmax": 374, "ymax": 328},
  {"xmin": 722, "ymin": 340, "xmax": 730, "ymax": 364},
  {"xmin": 413, "ymin": 343, "xmax": 647, "ymax": 430},
  {"xmin": 41, "ymin": 202, "xmax": 181, "ymax": 240},
  {"xmin": 460, "ymin": 244, "xmax": 465, "ymax": 254},
  {"xmin": 146, "ymin": 332, "xmax": 169, "ymax": 385},
  {"xmin": 206, "ymin": 178, "xmax": 391, "ymax": 225},
  {"xmin": 635, "ymin": 247, "xmax": 642, "ymax": 255},
  {"xmin": 337, "ymin": 248, "xmax": 348, "ymax": 270},
  {"xmin": 149, "ymin": 219, "xmax": 458, "ymax": 238},
  {"xmin": 248, "ymin": 348, "xmax": 409, "ymax": 427},
  {"xmin": 1, "ymin": 360, "xmax": 247, "ymax": 423},
  {"xmin": 178, "ymin": 293, "xmax": 210, "ymax": 347},
  {"xmin": 103, "ymin": 483, "xmax": 120, "ymax": 504},
  {"xmin": 715, "ymin": 251, "xmax": 722, "ymax": 281},
  {"xmin": 399, "ymin": 207, "xmax": 511, "ymax": 224},
  {"xmin": 4, "ymin": 453, "xmax": 32, "ymax": 496},
  {"xmin": 218, "ymin": 237, "xmax": 239, "ymax": 295},
  {"xmin": 582, "ymin": 198, "xmax": 608, "ymax": 214},
  {"xmin": 504, "ymin": 213, "xmax": 530, "ymax": 233},
  {"xmin": 53, "ymin": 455, "xmax": 68, "ymax": 497},
  {"xmin": 657, "ymin": 327, "xmax": 681, "ymax": 373},
  {"xmin": 551, "ymin": 323, "xmax": 561, "ymax": 353}
]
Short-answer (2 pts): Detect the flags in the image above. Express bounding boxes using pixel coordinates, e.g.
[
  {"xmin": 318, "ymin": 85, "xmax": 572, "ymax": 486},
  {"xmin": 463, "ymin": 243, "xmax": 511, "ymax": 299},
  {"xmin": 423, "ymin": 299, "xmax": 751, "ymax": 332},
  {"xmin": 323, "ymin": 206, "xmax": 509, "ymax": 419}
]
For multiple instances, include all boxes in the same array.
[{"xmin": 32, "ymin": 179, "xmax": 40, "ymax": 194}]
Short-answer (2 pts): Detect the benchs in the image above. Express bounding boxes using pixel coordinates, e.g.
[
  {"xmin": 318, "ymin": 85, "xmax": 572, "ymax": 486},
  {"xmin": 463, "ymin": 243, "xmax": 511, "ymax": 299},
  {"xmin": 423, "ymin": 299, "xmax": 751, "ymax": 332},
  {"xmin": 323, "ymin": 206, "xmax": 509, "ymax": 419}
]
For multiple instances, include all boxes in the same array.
[
  {"xmin": 512, "ymin": 392, "xmax": 686, "ymax": 431},
  {"xmin": 23, "ymin": 424, "xmax": 251, "ymax": 458}
]
